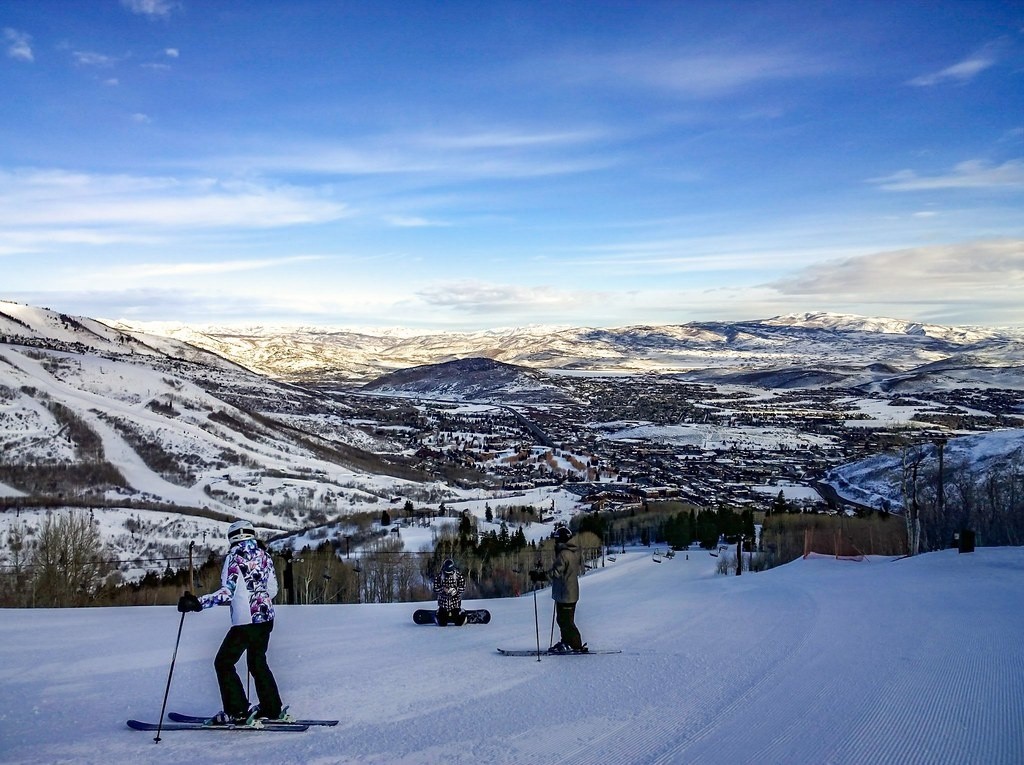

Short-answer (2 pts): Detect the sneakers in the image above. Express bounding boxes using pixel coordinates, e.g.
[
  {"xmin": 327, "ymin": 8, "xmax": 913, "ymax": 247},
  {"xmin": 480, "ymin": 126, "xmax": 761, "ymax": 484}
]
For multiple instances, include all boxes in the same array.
[
  {"xmin": 207, "ymin": 703, "xmax": 250, "ymax": 726},
  {"xmin": 248, "ymin": 703, "xmax": 282, "ymax": 720},
  {"xmin": 549, "ymin": 641, "xmax": 582, "ymax": 652}
]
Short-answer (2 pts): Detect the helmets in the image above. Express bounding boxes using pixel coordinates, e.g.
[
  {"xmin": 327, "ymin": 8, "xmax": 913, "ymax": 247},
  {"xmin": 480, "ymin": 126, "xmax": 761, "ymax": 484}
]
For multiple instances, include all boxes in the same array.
[
  {"xmin": 441, "ymin": 559, "xmax": 454, "ymax": 573},
  {"xmin": 553, "ymin": 527, "xmax": 572, "ymax": 544},
  {"xmin": 227, "ymin": 520, "xmax": 255, "ymax": 548}
]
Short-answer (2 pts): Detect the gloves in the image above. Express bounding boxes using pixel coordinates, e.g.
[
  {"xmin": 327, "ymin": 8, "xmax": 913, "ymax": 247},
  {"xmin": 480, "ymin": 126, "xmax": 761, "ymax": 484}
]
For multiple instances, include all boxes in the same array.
[
  {"xmin": 178, "ymin": 591, "xmax": 203, "ymax": 612},
  {"xmin": 446, "ymin": 587, "xmax": 457, "ymax": 596},
  {"xmin": 528, "ymin": 571, "xmax": 539, "ymax": 581}
]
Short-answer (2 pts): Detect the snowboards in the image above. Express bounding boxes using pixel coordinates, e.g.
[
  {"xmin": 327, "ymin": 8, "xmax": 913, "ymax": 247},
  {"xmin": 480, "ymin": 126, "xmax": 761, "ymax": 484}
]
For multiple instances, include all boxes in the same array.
[{"xmin": 412, "ymin": 608, "xmax": 491, "ymax": 625}]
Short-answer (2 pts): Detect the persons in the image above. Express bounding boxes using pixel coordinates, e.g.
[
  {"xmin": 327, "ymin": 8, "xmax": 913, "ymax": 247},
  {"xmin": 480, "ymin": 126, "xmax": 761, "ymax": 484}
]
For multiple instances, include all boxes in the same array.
[
  {"xmin": 528, "ymin": 525, "xmax": 588, "ymax": 653},
  {"xmin": 433, "ymin": 559, "xmax": 467, "ymax": 626},
  {"xmin": 178, "ymin": 519, "xmax": 281, "ymax": 727}
]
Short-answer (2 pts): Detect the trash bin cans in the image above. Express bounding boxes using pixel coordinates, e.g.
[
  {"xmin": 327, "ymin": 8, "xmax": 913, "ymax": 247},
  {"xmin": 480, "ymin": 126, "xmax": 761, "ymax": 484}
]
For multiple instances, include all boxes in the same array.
[{"xmin": 958, "ymin": 529, "xmax": 975, "ymax": 554}]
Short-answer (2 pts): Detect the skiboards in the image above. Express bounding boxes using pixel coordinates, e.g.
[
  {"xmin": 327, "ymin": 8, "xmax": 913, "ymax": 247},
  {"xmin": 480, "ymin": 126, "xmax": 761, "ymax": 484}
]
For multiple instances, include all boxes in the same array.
[
  {"xmin": 126, "ymin": 712, "xmax": 341, "ymax": 732},
  {"xmin": 496, "ymin": 647, "xmax": 623, "ymax": 657}
]
